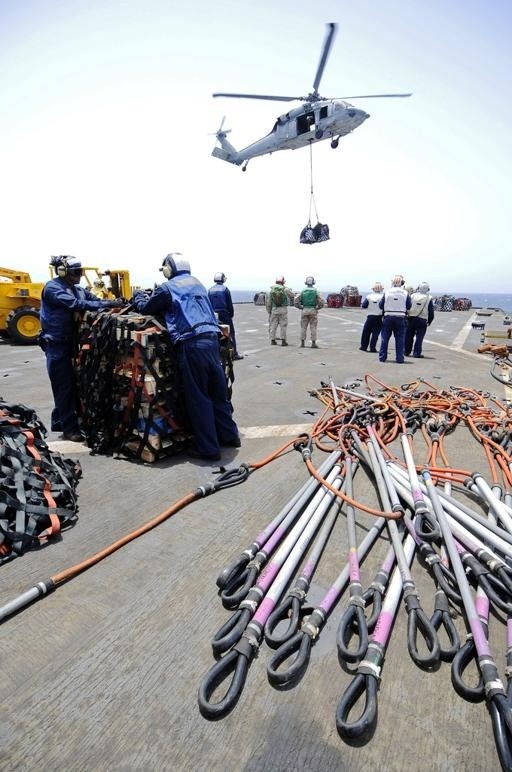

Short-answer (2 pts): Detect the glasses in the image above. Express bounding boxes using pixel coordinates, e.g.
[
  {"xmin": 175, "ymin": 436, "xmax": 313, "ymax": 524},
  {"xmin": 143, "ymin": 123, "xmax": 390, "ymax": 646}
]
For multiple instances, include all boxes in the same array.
[{"xmin": 69, "ymin": 269, "xmax": 83, "ymax": 276}]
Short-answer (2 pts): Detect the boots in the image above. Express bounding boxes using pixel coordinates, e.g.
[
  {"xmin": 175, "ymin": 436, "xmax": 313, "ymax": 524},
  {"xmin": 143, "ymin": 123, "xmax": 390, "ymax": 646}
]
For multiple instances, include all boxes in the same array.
[
  {"xmin": 271, "ymin": 340, "xmax": 277, "ymax": 345},
  {"xmin": 311, "ymin": 341, "xmax": 318, "ymax": 348},
  {"xmin": 281, "ymin": 340, "xmax": 288, "ymax": 346},
  {"xmin": 301, "ymin": 340, "xmax": 305, "ymax": 347}
]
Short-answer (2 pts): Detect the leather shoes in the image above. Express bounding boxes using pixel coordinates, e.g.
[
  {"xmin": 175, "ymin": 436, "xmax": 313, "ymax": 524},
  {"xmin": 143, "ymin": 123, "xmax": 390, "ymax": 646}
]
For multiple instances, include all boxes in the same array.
[
  {"xmin": 234, "ymin": 354, "xmax": 243, "ymax": 359},
  {"xmin": 218, "ymin": 438, "xmax": 241, "ymax": 447},
  {"xmin": 184, "ymin": 446, "xmax": 221, "ymax": 461},
  {"xmin": 63, "ymin": 431, "xmax": 84, "ymax": 442}
]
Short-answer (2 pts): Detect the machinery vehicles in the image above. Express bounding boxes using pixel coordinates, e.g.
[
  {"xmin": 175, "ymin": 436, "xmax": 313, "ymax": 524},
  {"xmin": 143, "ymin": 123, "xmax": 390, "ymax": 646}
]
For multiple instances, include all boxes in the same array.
[{"xmin": 0, "ymin": 264, "xmax": 133, "ymax": 346}]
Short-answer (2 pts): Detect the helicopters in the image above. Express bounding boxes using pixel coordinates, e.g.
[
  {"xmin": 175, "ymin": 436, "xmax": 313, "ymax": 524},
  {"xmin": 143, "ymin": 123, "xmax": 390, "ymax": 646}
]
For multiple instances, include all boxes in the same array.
[{"xmin": 210, "ymin": 20, "xmax": 414, "ymax": 175}]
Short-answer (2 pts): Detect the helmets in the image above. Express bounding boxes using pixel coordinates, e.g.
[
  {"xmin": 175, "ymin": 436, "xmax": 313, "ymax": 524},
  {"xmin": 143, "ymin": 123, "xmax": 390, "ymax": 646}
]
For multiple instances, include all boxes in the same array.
[
  {"xmin": 373, "ymin": 282, "xmax": 384, "ymax": 293},
  {"xmin": 214, "ymin": 272, "xmax": 227, "ymax": 283},
  {"xmin": 276, "ymin": 276, "xmax": 286, "ymax": 285},
  {"xmin": 390, "ymin": 275, "xmax": 405, "ymax": 287},
  {"xmin": 159, "ymin": 253, "xmax": 190, "ymax": 281},
  {"xmin": 54, "ymin": 256, "xmax": 83, "ymax": 284},
  {"xmin": 305, "ymin": 277, "xmax": 315, "ymax": 286},
  {"xmin": 418, "ymin": 282, "xmax": 430, "ymax": 293}
]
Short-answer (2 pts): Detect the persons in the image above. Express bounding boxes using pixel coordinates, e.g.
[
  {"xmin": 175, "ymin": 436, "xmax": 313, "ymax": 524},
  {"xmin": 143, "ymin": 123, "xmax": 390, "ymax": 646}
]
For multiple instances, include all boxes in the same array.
[
  {"xmin": 405, "ymin": 282, "xmax": 435, "ymax": 358},
  {"xmin": 358, "ymin": 282, "xmax": 383, "ymax": 352},
  {"xmin": 38, "ymin": 255, "xmax": 127, "ymax": 442},
  {"xmin": 89, "ymin": 279, "xmax": 112, "ymax": 300},
  {"xmin": 208, "ymin": 274, "xmax": 243, "ymax": 360},
  {"xmin": 379, "ymin": 276, "xmax": 412, "ymax": 363},
  {"xmin": 133, "ymin": 252, "xmax": 241, "ymax": 462},
  {"xmin": 293, "ymin": 277, "xmax": 323, "ymax": 349},
  {"xmin": 266, "ymin": 276, "xmax": 293, "ymax": 345}
]
